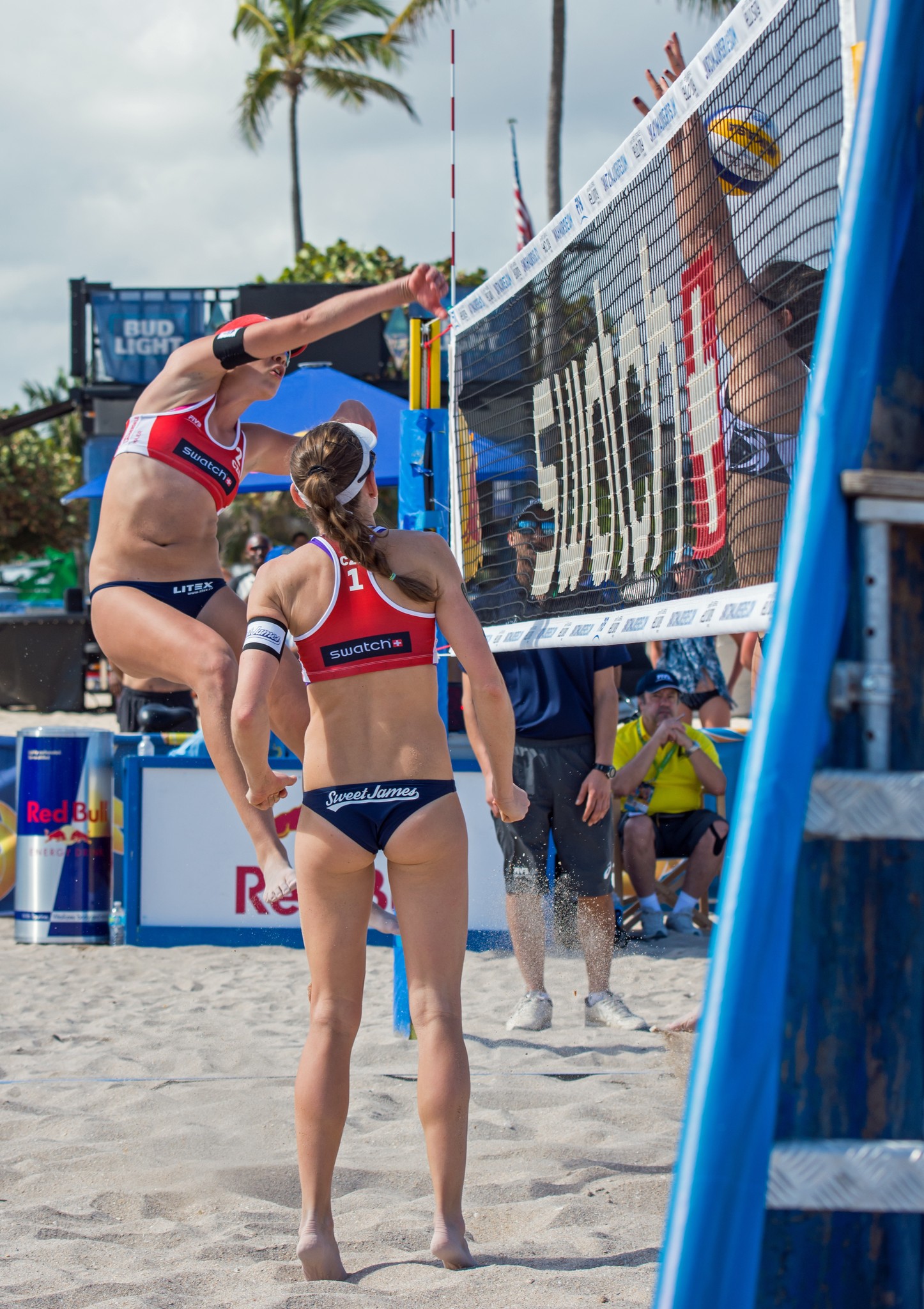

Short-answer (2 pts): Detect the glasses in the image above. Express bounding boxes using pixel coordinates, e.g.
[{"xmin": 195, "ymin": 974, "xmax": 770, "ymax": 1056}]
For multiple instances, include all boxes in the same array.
[
  {"xmin": 515, "ymin": 518, "xmax": 562, "ymax": 536},
  {"xmin": 358, "ymin": 450, "xmax": 376, "ymax": 483},
  {"xmin": 250, "ymin": 544, "xmax": 270, "ymax": 552},
  {"xmin": 671, "ymin": 560, "xmax": 697, "ymax": 571},
  {"xmin": 284, "ymin": 351, "xmax": 290, "ymax": 368}
]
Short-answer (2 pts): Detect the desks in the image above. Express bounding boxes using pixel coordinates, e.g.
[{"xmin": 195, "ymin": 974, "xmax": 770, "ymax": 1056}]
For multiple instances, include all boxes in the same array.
[{"xmin": 119, "ymin": 754, "xmax": 560, "ymax": 954}]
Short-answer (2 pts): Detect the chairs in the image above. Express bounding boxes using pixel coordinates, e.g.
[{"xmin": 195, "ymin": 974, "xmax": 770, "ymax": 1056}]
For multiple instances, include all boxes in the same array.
[{"xmin": 611, "ymin": 797, "xmax": 711, "ymax": 932}]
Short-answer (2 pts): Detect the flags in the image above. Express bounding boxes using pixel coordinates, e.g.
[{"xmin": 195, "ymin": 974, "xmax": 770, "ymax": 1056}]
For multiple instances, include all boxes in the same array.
[{"xmin": 514, "ymin": 186, "xmax": 533, "ymax": 252}]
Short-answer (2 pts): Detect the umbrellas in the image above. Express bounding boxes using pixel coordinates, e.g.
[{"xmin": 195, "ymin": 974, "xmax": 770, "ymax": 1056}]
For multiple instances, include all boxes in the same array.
[{"xmin": 61, "ymin": 363, "xmax": 534, "ymax": 506}]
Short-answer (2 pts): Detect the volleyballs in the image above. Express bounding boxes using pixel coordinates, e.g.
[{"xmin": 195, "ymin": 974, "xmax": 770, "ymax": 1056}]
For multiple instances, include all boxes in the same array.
[{"xmin": 703, "ymin": 104, "xmax": 782, "ymax": 196}]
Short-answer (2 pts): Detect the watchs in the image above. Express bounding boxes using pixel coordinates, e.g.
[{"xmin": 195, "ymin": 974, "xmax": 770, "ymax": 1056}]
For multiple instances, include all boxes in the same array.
[
  {"xmin": 593, "ymin": 762, "xmax": 617, "ymax": 779},
  {"xmin": 685, "ymin": 741, "xmax": 700, "ymax": 755}
]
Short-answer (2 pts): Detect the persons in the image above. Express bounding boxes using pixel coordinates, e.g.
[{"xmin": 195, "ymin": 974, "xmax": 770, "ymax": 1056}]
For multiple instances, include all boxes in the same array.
[
  {"xmin": 90, "ymin": 33, "xmax": 832, "ymax": 1030},
  {"xmin": 233, "ymin": 421, "xmax": 533, "ymax": 1280}
]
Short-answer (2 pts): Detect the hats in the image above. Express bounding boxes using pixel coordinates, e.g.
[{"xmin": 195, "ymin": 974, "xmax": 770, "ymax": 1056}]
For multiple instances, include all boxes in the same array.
[
  {"xmin": 635, "ymin": 669, "xmax": 683, "ymax": 697},
  {"xmin": 214, "ymin": 314, "xmax": 309, "ymax": 357},
  {"xmin": 290, "ymin": 422, "xmax": 377, "ymax": 509},
  {"xmin": 510, "ymin": 498, "xmax": 563, "ymax": 532},
  {"xmin": 264, "ymin": 546, "xmax": 295, "ymax": 564}
]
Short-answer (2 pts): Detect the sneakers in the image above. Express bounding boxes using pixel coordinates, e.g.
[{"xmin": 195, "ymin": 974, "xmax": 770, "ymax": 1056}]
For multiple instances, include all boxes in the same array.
[
  {"xmin": 665, "ymin": 907, "xmax": 703, "ymax": 936},
  {"xmin": 505, "ymin": 991, "xmax": 553, "ymax": 1031},
  {"xmin": 584, "ymin": 991, "xmax": 647, "ymax": 1030},
  {"xmin": 640, "ymin": 907, "xmax": 669, "ymax": 941}
]
